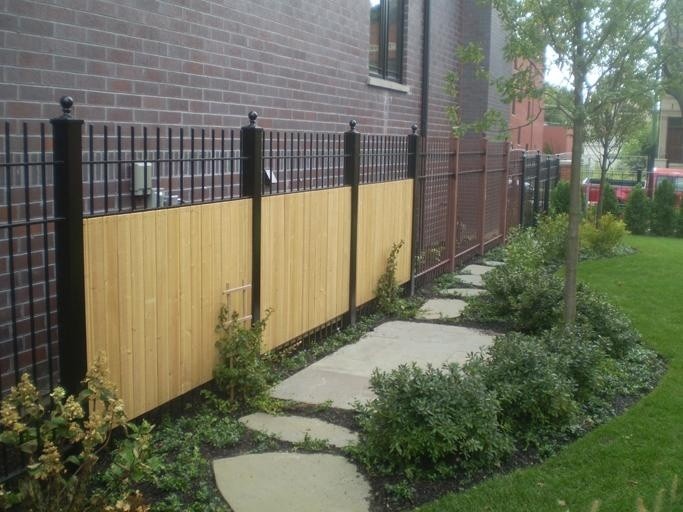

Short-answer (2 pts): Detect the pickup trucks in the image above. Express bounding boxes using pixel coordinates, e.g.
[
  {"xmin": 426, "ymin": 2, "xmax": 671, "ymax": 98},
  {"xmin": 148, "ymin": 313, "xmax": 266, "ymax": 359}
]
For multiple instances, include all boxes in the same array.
[{"xmin": 581, "ymin": 167, "xmax": 683, "ymax": 211}]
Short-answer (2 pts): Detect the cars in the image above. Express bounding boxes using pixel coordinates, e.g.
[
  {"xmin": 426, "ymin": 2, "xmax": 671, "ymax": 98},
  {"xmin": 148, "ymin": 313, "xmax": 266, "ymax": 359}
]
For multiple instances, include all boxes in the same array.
[{"xmin": 507, "ymin": 179, "xmax": 533, "ymax": 201}]
